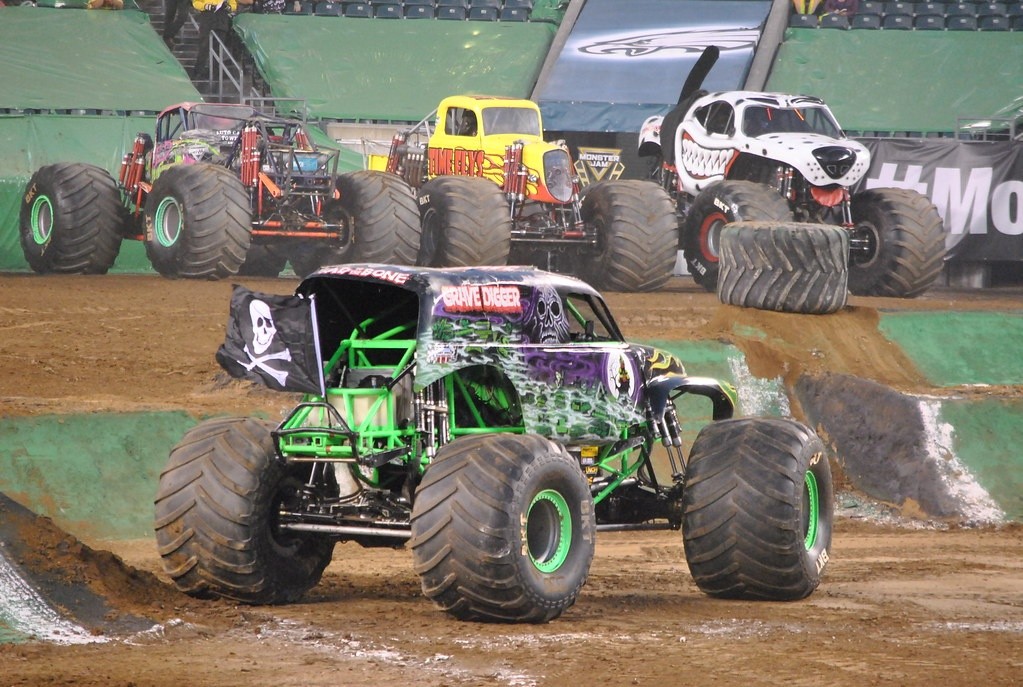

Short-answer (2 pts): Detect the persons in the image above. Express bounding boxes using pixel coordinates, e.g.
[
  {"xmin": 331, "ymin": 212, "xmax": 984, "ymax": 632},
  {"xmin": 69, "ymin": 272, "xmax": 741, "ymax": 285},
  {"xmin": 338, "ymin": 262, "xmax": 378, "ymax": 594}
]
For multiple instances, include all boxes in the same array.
[
  {"xmin": 163, "ymin": 0, "xmax": 302, "ymax": 80},
  {"xmin": 791, "ymin": 0, "xmax": 859, "ymax": 25},
  {"xmin": 460, "ymin": 110, "xmax": 477, "ymax": 135},
  {"xmin": 0, "ymin": 0, "xmax": 134, "ymax": 9}
]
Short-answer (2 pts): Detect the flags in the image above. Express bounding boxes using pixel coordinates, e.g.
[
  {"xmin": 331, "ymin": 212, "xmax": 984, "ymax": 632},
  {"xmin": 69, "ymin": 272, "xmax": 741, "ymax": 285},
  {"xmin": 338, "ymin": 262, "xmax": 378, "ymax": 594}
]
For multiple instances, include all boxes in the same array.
[{"xmin": 217, "ymin": 284, "xmax": 322, "ymax": 393}]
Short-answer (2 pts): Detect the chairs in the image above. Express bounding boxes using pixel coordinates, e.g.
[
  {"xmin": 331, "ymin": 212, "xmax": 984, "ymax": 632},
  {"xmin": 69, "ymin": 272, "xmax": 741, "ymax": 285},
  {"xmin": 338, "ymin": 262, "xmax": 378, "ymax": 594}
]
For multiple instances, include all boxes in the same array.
[
  {"xmin": 0, "ymin": 107, "xmax": 155, "ymax": 117},
  {"xmin": 341, "ymin": 0, "xmax": 533, "ymax": 17},
  {"xmin": 820, "ymin": 14, "xmax": 850, "ymax": 30},
  {"xmin": 407, "ymin": 6, "xmax": 434, "ymax": 19},
  {"xmin": 376, "ymin": 5, "xmax": 403, "ymax": 19},
  {"xmin": 500, "ymin": 7, "xmax": 528, "ymax": 22},
  {"xmin": 437, "ymin": 6, "xmax": 466, "ymax": 21},
  {"xmin": 790, "ymin": 13, "xmax": 818, "ymax": 29},
  {"xmin": 315, "ymin": 3, "xmax": 343, "ymax": 17},
  {"xmin": 346, "ymin": 4, "xmax": 373, "ymax": 18},
  {"xmin": 853, "ymin": 0, "xmax": 1023, "ymax": 31},
  {"xmin": 469, "ymin": 6, "xmax": 498, "ymax": 21},
  {"xmin": 284, "ymin": 2, "xmax": 313, "ymax": 16}
]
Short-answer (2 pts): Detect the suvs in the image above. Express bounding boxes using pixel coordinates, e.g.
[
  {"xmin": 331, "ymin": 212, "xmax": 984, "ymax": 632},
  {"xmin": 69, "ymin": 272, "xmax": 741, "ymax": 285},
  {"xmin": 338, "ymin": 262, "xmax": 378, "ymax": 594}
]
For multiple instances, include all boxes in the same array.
[
  {"xmin": 636, "ymin": 88, "xmax": 949, "ymax": 299},
  {"xmin": 152, "ymin": 262, "xmax": 836, "ymax": 623},
  {"xmin": 17, "ymin": 101, "xmax": 427, "ymax": 282},
  {"xmin": 365, "ymin": 90, "xmax": 678, "ymax": 289}
]
